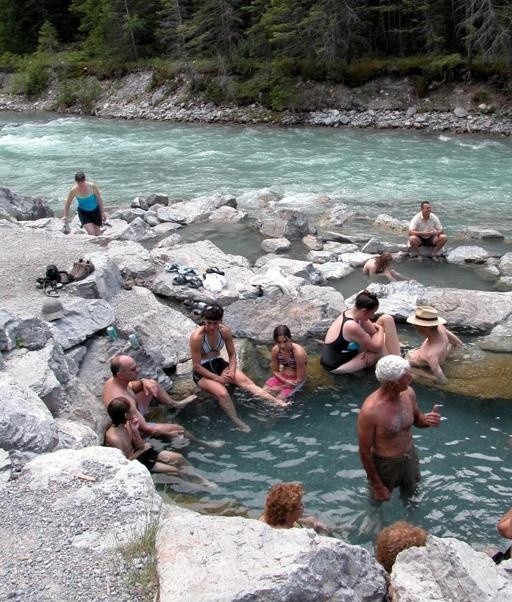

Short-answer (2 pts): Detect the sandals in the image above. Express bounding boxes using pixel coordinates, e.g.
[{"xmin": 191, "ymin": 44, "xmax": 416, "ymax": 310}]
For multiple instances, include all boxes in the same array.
[{"xmin": 35, "ymin": 277, "xmax": 62, "ymax": 290}]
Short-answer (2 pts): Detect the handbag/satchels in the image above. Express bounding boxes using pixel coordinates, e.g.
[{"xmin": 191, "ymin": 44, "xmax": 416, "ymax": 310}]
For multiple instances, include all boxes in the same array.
[{"xmin": 45, "ymin": 265, "xmax": 62, "ymax": 283}]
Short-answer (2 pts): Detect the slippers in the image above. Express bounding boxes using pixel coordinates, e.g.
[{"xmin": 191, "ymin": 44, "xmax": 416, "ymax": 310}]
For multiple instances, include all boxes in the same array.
[
  {"xmin": 123, "ymin": 270, "xmax": 147, "ymax": 290},
  {"xmin": 164, "ymin": 263, "xmax": 206, "ymax": 288},
  {"xmin": 206, "ymin": 267, "xmax": 224, "ymax": 275}
]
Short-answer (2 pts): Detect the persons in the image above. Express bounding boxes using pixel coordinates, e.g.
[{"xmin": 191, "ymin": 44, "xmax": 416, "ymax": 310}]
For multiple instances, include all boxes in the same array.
[
  {"xmin": 257, "ymin": 482, "xmax": 328, "ymax": 530},
  {"xmin": 104, "ymin": 396, "xmax": 218, "ymax": 492},
  {"xmin": 102, "ymin": 353, "xmax": 225, "ymax": 448},
  {"xmin": 61, "ymin": 172, "xmax": 107, "ymax": 235},
  {"xmin": 362, "ymin": 251, "xmax": 410, "ymax": 283},
  {"xmin": 407, "ymin": 201, "xmax": 447, "ymax": 262},
  {"xmin": 374, "ymin": 522, "xmax": 428, "ymax": 573},
  {"xmin": 376, "ymin": 306, "xmax": 463, "ymax": 385},
  {"xmin": 357, "ymin": 354, "xmax": 440, "ymax": 509},
  {"xmin": 190, "ymin": 304, "xmax": 293, "ymax": 433},
  {"xmin": 262, "ymin": 324, "xmax": 307, "ymax": 401},
  {"xmin": 321, "ymin": 290, "xmax": 388, "ymax": 375},
  {"xmin": 487, "ymin": 507, "xmax": 512, "ymax": 565}
]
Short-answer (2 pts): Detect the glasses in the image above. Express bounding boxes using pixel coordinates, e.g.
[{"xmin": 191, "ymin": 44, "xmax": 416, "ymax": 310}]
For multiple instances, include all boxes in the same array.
[{"xmin": 205, "ymin": 305, "xmax": 224, "ymax": 314}]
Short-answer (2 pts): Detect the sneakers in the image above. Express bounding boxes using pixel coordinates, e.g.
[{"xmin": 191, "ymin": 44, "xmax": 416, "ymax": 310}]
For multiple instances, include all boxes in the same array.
[{"xmin": 68, "ymin": 259, "xmax": 94, "ymax": 280}]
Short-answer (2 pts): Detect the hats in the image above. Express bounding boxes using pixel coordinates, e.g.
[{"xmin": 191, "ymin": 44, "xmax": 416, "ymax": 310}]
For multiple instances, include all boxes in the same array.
[{"xmin": 406, "ymin": 305, "xmax": 448, "ymax": 327}]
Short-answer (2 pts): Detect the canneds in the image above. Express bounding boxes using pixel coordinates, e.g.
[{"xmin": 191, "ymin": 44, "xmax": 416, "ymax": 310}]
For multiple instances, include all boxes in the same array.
[
  {"xmin": 107, "ymin": 327, "xmax": 117, "ymax": 339},
  {"xmin": 128, "ymin": 334, "xmax": 139, "ymax": 349}
]
